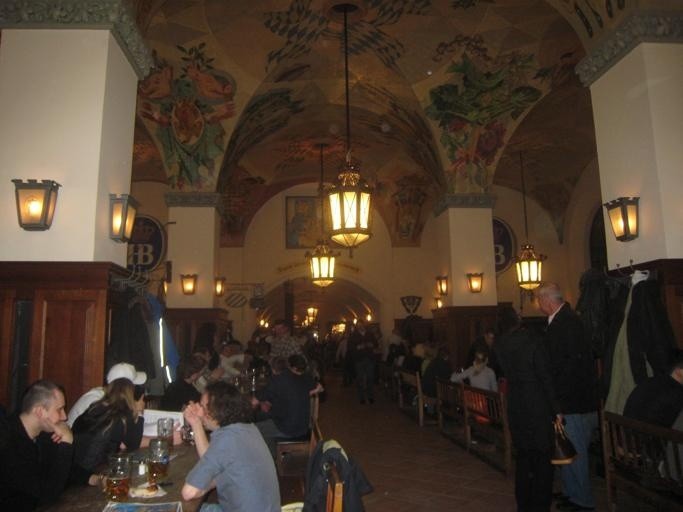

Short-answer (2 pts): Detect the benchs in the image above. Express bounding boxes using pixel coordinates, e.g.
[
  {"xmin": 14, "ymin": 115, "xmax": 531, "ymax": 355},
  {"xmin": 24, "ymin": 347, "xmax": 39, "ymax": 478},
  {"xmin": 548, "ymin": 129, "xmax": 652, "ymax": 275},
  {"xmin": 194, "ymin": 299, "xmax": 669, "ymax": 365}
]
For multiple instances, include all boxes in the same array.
[
  {"xmin": 436, "ymin": 375, "xmax": 511, "ymax": 483},
  {"xmin": 598, "ymin": 409, "xmax": 681, "ymax": 510},
  {"xmin": 372, "ymin": 357, "xmax": 464, "ymax": 429}
]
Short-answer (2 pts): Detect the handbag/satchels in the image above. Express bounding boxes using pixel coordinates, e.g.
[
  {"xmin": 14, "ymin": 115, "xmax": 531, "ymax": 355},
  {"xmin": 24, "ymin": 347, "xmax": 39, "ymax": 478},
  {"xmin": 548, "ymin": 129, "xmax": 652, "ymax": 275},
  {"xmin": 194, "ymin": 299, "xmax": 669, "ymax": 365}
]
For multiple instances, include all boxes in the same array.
[{"xmin": 549, "ymin": 418, "xmax": 580, "ymax": 466}]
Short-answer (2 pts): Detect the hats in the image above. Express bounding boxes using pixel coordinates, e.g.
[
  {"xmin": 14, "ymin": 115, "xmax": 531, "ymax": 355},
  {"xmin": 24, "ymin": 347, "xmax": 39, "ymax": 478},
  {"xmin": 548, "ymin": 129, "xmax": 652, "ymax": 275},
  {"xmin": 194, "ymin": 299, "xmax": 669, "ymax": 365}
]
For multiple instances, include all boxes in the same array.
[{"xmin": 105, "ymin": 361, "xmax": 148, "ymax": 387}]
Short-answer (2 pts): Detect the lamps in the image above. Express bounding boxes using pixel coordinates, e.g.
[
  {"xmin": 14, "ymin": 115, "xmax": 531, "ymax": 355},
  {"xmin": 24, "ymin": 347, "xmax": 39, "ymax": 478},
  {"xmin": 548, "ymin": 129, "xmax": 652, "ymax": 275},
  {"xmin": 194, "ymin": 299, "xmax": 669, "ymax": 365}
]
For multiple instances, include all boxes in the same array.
[
  {"xmin": 304, "ymin": 238, "xmax": 341, "ymax": 290},
  {"xmin": 435, "ymin": 275, "xmax": 448, "ymax": 296},
  {"xmin": 466, "ymin": 272, "xmax": 484, "ymax": 293},
  {"xmin": 179, "ymin": 274, "xmax": 198, "ymax": 295},
  {"xmin": 11, "ymin": 178, "xmax": 63, "ymax": 231},
  {"xmin": 108, "ymin": 193, "xmax": 141, "ymax": 243},
  {"xmin": 259, "ymin": 304, "xmax": 373, "ymax": 336},
  {"xmin": 322, "ymin": 2, "xmax": 376, "ymax": 261},
  {"xmin": 512, "ymin": 148, "xmax": 548, "ymax": 298},
  {"xmin": 603, "ymin": 197, "xmax": 641, "ymax": 242},
  {"xmin": 215, "ymin": 275, "xmax": 226, "ymax": 297}
]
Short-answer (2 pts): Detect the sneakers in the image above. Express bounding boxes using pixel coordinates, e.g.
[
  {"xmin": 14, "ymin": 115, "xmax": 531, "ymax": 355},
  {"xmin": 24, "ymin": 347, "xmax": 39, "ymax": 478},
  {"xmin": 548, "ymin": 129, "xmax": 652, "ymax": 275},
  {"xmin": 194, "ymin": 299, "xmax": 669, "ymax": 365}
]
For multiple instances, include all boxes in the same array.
[
  {"xmin": 359, "ymin": 397, "xmax": 376, "ymax": 405},
  {"xmin": 551, "ymin": 492, "xmax": 595, "ymax": 511}
]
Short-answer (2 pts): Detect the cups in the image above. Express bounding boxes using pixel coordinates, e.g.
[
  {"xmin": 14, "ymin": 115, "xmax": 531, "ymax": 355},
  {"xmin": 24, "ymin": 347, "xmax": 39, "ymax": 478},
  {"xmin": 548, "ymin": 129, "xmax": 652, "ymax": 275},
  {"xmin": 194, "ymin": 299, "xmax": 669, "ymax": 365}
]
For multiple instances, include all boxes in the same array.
[
  {"xmin": 105, "ymin": 451, "xmax": 131, "ymax": 502},
  {"xmin": 148, "ymin": 440, "xmax": 169, "ymax": 474},
  {"xmin": 157, "ymin": 418, "xmax": 174, "ymax": 447}
]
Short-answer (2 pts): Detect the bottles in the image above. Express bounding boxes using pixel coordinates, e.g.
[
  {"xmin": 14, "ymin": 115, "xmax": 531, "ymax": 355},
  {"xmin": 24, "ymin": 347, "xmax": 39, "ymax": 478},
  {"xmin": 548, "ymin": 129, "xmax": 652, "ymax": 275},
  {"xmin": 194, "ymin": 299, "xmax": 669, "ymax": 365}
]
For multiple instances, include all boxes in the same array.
[
  {"xmin": 137, "ymin": 459, "xmax": 145, "ymax": 475},
  {"xmin": 250, "ymin": 375, "xmax": 257, "ymax": 392},
  {"xmin": 235, "ymin": 377, "xmax": 240, "ymax": 388}
]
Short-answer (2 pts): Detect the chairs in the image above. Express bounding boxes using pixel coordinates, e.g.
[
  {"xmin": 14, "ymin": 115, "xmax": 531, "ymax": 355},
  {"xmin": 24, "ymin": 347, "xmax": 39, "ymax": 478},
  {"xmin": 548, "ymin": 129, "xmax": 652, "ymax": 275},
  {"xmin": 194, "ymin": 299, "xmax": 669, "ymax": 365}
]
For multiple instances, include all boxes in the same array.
[{"xmin": 142, "ymin": 380, "xmax": 344, "ymax": 512}]
